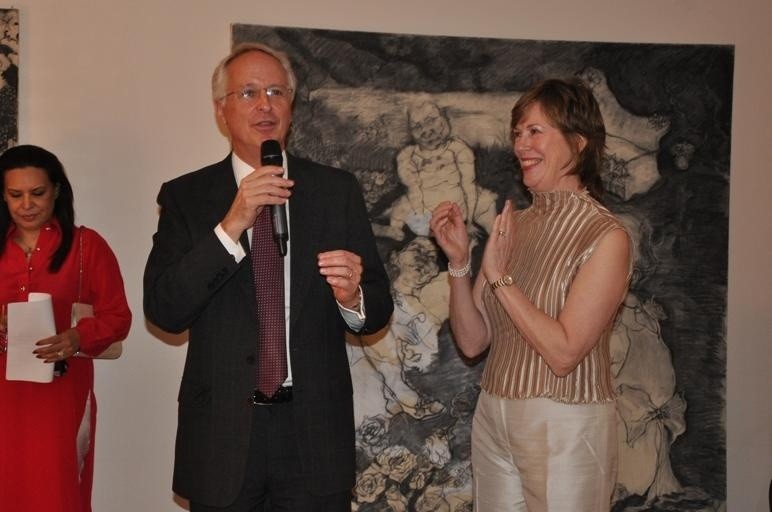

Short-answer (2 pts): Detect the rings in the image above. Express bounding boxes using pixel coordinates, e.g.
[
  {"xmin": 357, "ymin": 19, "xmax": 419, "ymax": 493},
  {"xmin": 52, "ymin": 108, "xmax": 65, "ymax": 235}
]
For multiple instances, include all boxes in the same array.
[
  {"xmin": 347, "ymin": 269, "xmax": 354, "ymax": 279},
  {"xmin": 496, "ymin": 237, "xmax": 505, "ymax": 241},
  {"xmin": 56, "ymin": 349, "xmax": 65, "ymax": 358},
  {"xmin": 497, "ymin": 230, "xmax": 506, "ymax": 237}
]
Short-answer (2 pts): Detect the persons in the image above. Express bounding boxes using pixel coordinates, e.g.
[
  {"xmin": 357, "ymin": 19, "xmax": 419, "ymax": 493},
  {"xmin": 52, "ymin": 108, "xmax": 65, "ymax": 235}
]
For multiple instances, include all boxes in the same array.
[
  {"xmin": 140, "ymin": 41, "xmax": 395, "ymax": 511},
  {"xmin": 358, "ymin": 233, "xmax": 449, "ymax": 422},
  {"xmin": 346, "ymin": 232, "xmax": 441, "ymax": 366},
  {"xmin": 426, "ymin": 75, "xmax": 639, "ymax": 512},
  {"xmin": 0, "ymin": 142, "xmax": 133, "ymax": 511},
  {"xmin": 400, "ymin": 87, "xmax": 513, "ymax": 243}
]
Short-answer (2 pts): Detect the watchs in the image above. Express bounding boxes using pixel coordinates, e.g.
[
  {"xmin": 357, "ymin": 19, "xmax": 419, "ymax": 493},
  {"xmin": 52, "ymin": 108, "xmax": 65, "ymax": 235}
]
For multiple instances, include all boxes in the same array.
[
  {"xmin": 71, "ymin": 347, "xmax": 80, "ymax": 358},
  {"xmin": 350, "ymin": 288, "xmax": 363, "ymax": 314},
  {"xmin": 489, "ymin": 271, "xmax": 516, "ymax": 293}
]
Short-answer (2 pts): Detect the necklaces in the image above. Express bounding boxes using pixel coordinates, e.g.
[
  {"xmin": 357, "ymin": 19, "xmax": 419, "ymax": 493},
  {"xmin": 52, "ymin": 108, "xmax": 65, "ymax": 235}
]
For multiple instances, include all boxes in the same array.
[{"xmin": 13, "ymin": 230, "xmax": 41, "ymax": 259}]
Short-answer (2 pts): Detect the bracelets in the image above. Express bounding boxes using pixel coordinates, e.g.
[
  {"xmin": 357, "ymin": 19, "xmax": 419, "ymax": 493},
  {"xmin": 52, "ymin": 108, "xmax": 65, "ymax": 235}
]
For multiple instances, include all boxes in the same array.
[{"xmin": 446, "ymin": 259, "xmax": 471, "ymax": 278}]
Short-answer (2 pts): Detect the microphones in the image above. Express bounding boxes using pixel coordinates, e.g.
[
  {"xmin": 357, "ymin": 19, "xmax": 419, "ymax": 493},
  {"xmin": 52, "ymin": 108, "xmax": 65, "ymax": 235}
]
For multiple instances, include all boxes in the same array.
[{"xmin": 259, "ymin": 138, "xmax": 295, "ymax": 259}]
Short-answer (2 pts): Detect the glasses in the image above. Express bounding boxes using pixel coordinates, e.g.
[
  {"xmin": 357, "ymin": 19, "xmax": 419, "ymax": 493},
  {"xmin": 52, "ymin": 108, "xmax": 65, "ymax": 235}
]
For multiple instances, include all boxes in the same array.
[{"xmin": 216, "ymin": 83, "xmax": 293, "ymax": 106}]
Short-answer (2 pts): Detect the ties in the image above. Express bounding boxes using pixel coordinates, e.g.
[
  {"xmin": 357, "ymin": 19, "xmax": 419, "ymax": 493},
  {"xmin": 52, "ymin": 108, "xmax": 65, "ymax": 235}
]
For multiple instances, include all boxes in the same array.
[{"xmin": 251, "ymin": 205, "xmax": 288, "ymax": 398}]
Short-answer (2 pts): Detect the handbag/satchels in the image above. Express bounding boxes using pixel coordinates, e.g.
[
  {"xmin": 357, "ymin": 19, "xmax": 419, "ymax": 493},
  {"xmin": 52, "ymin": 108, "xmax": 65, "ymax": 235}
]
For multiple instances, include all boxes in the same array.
[{"xmin": 70, "ymin": 225, "xmax": 123, "ymax": 361}]
